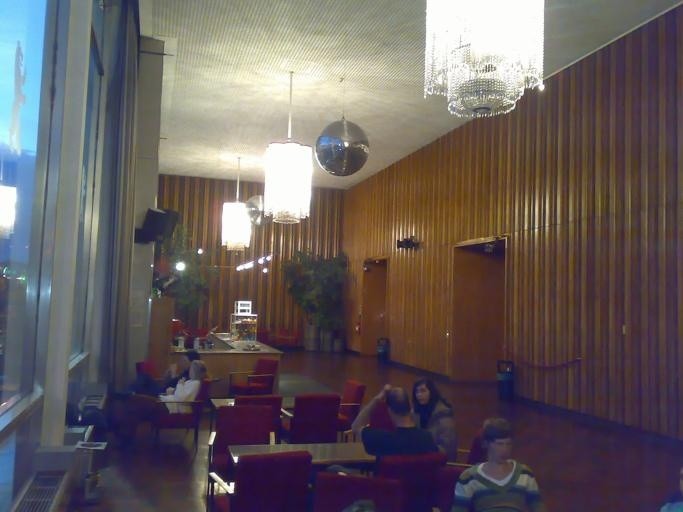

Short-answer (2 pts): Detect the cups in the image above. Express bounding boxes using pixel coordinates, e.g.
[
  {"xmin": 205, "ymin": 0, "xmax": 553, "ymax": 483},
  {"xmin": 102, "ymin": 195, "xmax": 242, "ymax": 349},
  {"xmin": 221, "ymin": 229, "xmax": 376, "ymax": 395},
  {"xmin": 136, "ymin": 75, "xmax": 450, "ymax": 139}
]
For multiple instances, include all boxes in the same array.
[{"xmin": 177, "ymin": 337, "xmax": 184, "ymax": 351}]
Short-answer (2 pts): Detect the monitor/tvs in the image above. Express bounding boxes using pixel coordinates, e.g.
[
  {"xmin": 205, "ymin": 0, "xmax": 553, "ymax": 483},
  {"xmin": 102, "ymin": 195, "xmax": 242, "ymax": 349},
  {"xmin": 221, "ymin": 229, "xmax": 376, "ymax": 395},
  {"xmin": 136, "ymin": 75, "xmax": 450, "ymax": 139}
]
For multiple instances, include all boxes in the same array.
[{"xmin": 141, "ymin": 207, "xmax": 179, "ymax": 242}]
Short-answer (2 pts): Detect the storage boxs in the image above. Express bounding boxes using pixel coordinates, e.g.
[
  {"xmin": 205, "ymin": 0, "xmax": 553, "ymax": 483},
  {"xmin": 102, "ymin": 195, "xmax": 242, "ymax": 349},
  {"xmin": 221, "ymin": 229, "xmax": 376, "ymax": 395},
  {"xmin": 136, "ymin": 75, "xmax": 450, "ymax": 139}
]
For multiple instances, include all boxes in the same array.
[{"xmin": 314, "ymin": 76, "xmax": 370, "ymax": 176}]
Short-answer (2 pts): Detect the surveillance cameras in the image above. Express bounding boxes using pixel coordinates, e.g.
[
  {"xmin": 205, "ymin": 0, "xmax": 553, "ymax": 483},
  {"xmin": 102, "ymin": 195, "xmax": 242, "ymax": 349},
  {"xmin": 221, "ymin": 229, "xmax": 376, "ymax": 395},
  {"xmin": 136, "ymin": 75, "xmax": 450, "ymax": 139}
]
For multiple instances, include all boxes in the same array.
[{"xmin": 396, "ymin": 237, "xmax": 415, "ymax": 250}]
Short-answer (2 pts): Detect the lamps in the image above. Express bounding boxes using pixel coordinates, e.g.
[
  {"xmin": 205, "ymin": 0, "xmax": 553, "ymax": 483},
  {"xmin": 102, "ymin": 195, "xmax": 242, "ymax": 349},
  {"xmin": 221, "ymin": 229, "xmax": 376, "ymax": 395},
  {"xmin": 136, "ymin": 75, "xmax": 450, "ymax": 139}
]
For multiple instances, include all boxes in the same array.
[
  {"xmin": 423, "ymin": 1, "xmax": 545, "ymax": 119},
  {"xmin": 398, "ymin": 238, "xmax": 416, "ymax": 249},
  {"xmin": 221, "ymin": 156, "xmax": 252, "ymax": 250},
  {"xmin": 261, "ymin": 71, "xmax": 317, "ymax": 223},
  {"xmin": 244, "ymin": 192, "xmax": 265, "ymax": 225}
]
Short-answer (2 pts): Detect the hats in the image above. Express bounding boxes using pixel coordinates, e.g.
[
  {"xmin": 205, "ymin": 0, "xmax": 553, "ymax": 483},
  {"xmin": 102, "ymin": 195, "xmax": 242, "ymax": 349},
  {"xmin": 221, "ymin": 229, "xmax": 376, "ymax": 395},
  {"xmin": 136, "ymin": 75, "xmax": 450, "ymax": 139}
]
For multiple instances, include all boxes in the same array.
[{"xmin": 483, "ymin": 418, "xmax": 520, "ymax": 439}]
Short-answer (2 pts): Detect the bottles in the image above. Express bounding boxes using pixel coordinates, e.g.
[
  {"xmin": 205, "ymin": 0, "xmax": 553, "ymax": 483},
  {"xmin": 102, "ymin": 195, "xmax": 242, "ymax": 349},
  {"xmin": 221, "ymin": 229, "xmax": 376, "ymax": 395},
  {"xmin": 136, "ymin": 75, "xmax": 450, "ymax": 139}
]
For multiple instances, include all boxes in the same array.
[{"xmin": 193, "ymin": 337, "xmax": 199, "ymax": 352}]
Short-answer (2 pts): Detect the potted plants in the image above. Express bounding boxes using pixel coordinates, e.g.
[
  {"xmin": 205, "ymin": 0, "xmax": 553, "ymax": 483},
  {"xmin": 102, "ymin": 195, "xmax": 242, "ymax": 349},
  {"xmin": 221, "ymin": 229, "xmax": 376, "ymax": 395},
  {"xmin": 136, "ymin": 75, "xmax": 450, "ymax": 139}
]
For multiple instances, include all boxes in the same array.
[
  {"xmin": 278, "ymin": 247, "xmax": 349, "ymax": 353},
  {"xmin": 153, "ymin": 223, "xmax": 219, "ymax": 348}
]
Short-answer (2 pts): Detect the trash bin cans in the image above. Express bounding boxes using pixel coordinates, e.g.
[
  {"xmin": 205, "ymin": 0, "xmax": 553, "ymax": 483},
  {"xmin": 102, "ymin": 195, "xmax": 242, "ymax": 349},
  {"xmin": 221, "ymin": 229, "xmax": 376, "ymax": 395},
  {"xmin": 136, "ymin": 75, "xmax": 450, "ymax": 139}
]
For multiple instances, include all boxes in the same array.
[
  {"xmin": 496, "ymin": 361, "xmax": 514, "ymax": 403},
  {"xmin": 377, "ymin": 336, "xmax": 390, "ymax": 365},
  {"xmin": 334, "ymin": 327, "xmax": 346, "ymax": 352}
]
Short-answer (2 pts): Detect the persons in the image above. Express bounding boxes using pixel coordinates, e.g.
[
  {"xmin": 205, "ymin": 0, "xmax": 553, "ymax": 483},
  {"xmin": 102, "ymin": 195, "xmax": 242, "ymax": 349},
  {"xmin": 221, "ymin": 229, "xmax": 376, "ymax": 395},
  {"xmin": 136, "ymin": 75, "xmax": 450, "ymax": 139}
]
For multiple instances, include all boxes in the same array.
[
  {"xmin": 325, "ymin": 383, "xmax": 440, "ymax": 476},
  {"xmin": 448, "ymin": 415, "xmax": 546, "ymax": 512},
  {"xmin": 410, "ymin": 378, "xmax": 463, "ymax": 464},
  {"xmin": 109, "ymin": 351, "xmax": 201, "ymax": 403},
  {"xmin": 657, "ymin": 465, "xmax": 683, "ymax": 511},
  {"xmin": 115, "ymin": 358, "xmax": 208, "ymax": 444}
]
